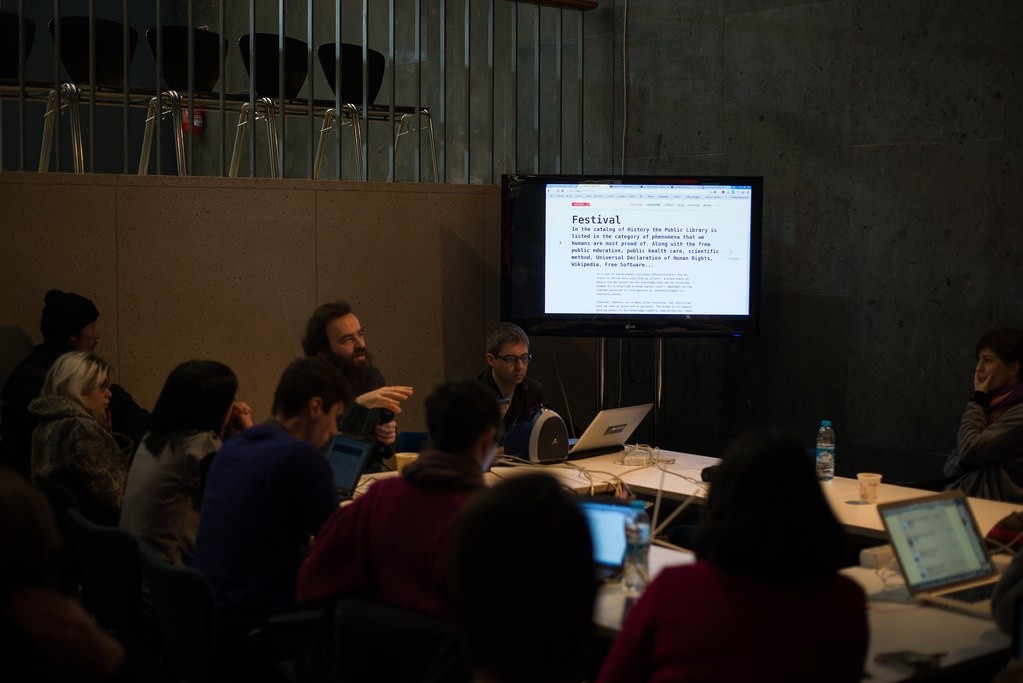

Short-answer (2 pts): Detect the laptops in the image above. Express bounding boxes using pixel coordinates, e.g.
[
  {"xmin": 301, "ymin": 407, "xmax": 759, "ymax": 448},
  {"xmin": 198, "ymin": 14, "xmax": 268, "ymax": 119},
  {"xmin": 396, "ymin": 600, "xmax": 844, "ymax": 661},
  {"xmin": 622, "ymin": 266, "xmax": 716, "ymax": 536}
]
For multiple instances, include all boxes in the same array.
[
  {"xmin": 569, "ymin": 403, "xmax": 652, "ymax": 455},
  {"xmin": 575, "ymin": 495, "xmax": 644, "ymax": 583},
  {"xmin": 322, "ymin": 429, "xmax": 378, "ymax": 500},
  {"xmin": 875, "ymin": 490, "xmax": 1005, "ymax": 620}
]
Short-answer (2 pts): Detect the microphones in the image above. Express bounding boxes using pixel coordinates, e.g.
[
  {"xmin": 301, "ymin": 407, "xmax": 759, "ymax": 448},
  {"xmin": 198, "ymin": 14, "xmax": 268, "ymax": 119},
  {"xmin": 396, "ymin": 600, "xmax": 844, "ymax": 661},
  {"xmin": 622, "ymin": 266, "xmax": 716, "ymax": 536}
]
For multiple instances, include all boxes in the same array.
[{"xmin": 375, "ymin": 409, "xmax": 395, "ymax": 456}]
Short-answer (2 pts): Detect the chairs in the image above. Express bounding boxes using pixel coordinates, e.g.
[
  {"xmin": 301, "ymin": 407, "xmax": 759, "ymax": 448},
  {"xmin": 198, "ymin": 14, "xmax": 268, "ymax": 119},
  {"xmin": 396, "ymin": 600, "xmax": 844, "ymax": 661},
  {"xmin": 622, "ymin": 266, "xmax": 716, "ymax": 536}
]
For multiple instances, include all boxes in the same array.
[
  {"xmin": 44, "ymin": 484, "xmax": 467, "ymax": 683},
  {"xmin": 0, "ymin": 12, "xmax": 439, "ymax": 184}
]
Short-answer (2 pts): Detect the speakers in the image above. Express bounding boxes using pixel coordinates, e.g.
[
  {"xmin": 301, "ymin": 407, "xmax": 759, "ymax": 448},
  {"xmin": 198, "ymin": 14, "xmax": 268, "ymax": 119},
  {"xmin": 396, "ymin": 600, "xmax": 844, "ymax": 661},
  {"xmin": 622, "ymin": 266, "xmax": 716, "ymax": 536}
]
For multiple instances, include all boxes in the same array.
[{"xmin": 503, "ymin": 404, "xmax": 569, "ymax": 464}]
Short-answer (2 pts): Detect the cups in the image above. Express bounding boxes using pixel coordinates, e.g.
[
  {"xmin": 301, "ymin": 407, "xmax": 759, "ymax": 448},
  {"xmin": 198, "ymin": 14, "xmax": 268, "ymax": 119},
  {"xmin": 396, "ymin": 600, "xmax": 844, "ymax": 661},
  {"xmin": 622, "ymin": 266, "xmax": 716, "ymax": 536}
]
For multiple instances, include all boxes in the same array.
[
  {"xmin": 396, "ymin": 452, "xmax": 420, "ymax": 476},
  {"xmin": 855, "ymin": 473, "xmax": 883, "ymax": 505}
]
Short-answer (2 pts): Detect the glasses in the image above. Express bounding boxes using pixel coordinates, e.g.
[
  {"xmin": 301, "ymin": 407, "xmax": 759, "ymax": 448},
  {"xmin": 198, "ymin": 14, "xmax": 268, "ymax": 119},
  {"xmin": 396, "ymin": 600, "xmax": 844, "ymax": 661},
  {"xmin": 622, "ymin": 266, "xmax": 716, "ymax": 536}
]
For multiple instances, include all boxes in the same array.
[
  {"xmin": 494, "ymin": 353, "xmax": 536, "ymax": 364},
  {"xmin": 89, "ymin": 379, "xmax": 110, "ymax": 392}
]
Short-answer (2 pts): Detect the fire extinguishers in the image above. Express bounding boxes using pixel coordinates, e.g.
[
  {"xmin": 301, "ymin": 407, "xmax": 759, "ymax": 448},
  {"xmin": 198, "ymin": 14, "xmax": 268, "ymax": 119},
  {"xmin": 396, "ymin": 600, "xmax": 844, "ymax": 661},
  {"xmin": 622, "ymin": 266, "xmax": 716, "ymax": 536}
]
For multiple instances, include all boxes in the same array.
[{"xmin": 181, "ymin": 109, "xmax": 203, "ymax": 135}]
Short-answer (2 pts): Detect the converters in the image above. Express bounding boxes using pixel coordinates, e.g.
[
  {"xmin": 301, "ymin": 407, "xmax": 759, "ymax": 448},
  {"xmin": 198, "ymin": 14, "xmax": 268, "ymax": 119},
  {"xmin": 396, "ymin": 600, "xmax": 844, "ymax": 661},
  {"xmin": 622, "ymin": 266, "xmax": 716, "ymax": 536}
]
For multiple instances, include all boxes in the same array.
[{"xmin": 624, "ymin": 456, "xmax": 650, "ymax": 465}]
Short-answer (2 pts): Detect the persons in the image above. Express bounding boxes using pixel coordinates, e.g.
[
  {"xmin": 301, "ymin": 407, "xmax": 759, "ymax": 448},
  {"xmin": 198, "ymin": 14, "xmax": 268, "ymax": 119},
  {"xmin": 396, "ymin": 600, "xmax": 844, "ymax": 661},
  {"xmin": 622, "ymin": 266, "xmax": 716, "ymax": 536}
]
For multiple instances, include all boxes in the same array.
[
  {"xmin": 190, "ymin": 356, "xmax": 354, "ymax": 617},
  {"xmin": 0, "ymin": 466, "xmax": 169, "ymax": 683},
  {"xmin": 596, "ymin": 432, "xmax": 870, "ymax": 683},
  {"xmin": 458, "ymin": 473, "xmax": 596, "ymax": 683},
  {"xmin": 298, "ymin": 302, "xmax": 412, "ymax": 468},
  {"xmin": 296, "ymin": 382, "xmax": 500, "ymax": 621},
  {"xmin": 933, "ymin": 327, "xmax": 1023, "ymax": 683},
  {"xmin": 472, "ymin": 322, "xmax": 549, "ymax": 464},
  {"xmin": 120, "ymin": 361, "xmax": 255, "ymax": 566},
  {"xmin": 0, "ymin": 289, "xmax": 150, "ymax": 478},
  {"xmin": 29, "ymin": 351, "xmax": 128, "ymax": 511}
]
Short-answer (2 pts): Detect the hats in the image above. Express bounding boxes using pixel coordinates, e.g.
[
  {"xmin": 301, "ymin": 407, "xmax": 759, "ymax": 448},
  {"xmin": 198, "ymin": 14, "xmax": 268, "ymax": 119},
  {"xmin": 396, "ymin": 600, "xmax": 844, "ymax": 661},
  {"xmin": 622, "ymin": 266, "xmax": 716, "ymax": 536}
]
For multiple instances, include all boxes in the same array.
[{"xmin": 41, "ymin": 289, "xmax": 99, "ymax": 345}]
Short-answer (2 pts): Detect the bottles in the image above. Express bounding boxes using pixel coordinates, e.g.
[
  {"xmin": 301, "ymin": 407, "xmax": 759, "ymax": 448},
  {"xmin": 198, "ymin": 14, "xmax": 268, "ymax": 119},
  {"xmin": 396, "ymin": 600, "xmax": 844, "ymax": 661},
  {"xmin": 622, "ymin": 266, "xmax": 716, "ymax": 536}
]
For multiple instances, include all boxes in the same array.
[
  {"xmin": 624, "ymin": 501, "xmax": 650, "ymax": 595},
  {"xmin": 816, "ymin": 420, "xmax": 836, "ymax": 481}
]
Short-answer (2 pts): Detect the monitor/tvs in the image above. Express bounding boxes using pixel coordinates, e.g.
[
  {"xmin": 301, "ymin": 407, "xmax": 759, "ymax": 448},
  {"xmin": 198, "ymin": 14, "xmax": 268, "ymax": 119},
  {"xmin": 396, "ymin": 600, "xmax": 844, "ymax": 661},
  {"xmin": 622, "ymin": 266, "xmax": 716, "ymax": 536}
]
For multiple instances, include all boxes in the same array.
[{"xmin": 500, "ymin": 175, "xmax": 764, "ymax": 339}]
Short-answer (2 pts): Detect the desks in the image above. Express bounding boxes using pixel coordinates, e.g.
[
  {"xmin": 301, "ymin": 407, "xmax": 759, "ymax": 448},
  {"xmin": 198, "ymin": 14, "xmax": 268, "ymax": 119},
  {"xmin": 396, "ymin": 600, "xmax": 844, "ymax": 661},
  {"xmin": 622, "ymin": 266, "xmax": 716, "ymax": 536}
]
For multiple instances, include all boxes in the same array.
[{"xmin": 338, "ymin": 444, "xmax": 1023, "ymax": 683}]
